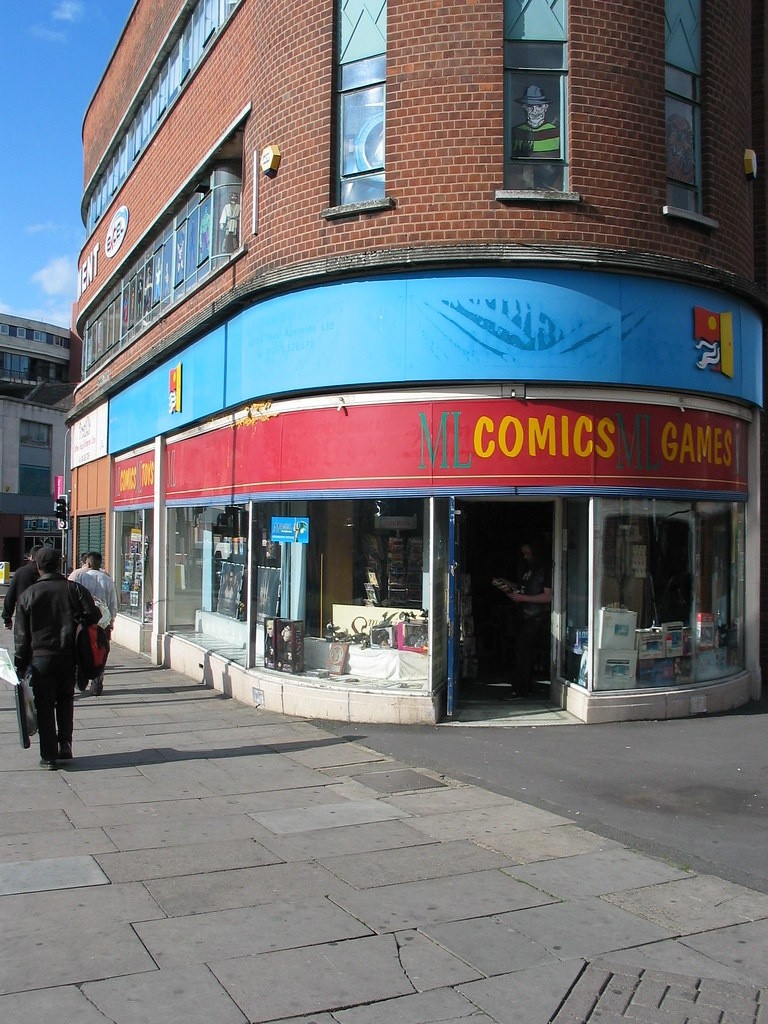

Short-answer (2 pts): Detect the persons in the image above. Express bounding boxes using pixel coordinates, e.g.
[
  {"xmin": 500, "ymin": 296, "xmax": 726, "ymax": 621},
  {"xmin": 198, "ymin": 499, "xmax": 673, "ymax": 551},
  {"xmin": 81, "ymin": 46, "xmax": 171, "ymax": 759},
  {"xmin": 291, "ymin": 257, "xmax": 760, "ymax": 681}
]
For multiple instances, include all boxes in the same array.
[
  {"xmin": 223, "ymin": 570, "xmax": 236, "ymax": 598},
  {"xmin": 499, "ymin": 532, "xmax": 552, "ymax": 700},
  {"xmin": 2, "ymin": 542, "xmax": 45, "ymax": 678},
  {"xmin": 67, "ymin": 549, "xmax": 122, "ymax": 696},
  {"xmin": 14, "ymin": 546, "xmax": 96, "ymax": 769}
]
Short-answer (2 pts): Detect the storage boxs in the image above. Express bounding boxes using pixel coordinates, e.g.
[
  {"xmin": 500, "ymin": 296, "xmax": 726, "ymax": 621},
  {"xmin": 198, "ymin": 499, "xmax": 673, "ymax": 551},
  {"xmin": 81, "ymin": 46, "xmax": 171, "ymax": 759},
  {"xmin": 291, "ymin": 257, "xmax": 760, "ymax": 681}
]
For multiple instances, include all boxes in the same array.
[
  {"xmin": 397, "ymin": 619, "xmax": 429, "ymax": 653},
  {"xmin": 370, "ymin": 625, "xmax": 398, "ymax": 649},
  {"xmin": 591, "ymin": 609, "xmax": 728, "ymax": 691}
]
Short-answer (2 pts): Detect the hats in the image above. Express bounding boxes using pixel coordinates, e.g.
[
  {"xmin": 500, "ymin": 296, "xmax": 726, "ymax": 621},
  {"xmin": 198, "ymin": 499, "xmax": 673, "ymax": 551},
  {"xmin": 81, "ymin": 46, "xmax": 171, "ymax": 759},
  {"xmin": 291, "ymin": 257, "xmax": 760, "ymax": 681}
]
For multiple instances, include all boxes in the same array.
[{"xmin": 35, "ymin": 546, "xmax": 58, "ymax": 567}]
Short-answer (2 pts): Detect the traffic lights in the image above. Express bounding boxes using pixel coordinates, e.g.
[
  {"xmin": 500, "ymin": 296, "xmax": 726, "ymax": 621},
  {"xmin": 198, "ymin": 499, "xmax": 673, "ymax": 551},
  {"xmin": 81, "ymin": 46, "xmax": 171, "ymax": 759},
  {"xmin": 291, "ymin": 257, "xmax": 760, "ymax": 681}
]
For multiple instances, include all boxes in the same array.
[{"xmin": 56, "ymin": 494, "xmax": 69, "ymax": 529}]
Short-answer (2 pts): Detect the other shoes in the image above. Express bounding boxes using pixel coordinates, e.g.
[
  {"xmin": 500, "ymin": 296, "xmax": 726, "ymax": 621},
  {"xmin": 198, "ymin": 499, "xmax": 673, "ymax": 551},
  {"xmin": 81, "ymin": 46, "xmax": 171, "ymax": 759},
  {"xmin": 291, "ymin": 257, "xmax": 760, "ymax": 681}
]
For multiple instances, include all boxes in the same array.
[
  {"xmin": 56, "ymin": 743, "xmax": 72, "ymax": 759},
  {"xmin": 39, "ymin": 757, "xmax": 58, "ymax": 770},
  {"xmin": 502, "ymin": 686, "xmax": 533, "ymax": 701},
  {"xmin": 90, "ymin": 684, "xmax": 103, "ymax": 696}
]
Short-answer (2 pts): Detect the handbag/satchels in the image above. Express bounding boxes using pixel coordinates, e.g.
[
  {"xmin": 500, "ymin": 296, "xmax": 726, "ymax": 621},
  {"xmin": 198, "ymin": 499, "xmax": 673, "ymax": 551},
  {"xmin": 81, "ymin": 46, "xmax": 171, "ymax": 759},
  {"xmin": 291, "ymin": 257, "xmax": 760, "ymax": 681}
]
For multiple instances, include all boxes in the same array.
[
  {"xmin": 14, "ymin": 677, "xmax": 38, "ymax": 748},
  {"xmin": 76, "ymin": 625, "xmax": 108, "ymax": 679}
]
